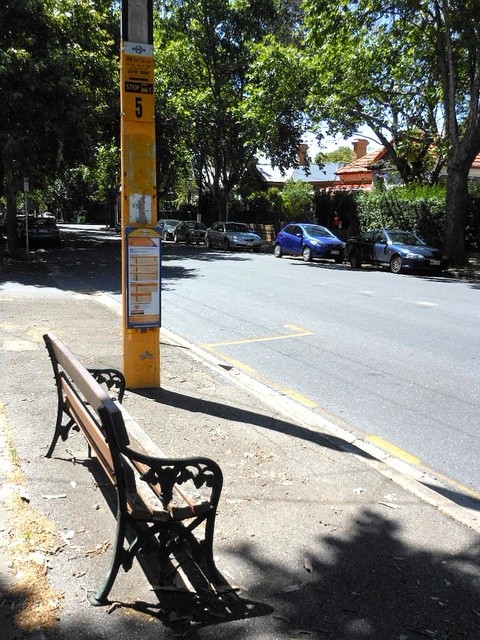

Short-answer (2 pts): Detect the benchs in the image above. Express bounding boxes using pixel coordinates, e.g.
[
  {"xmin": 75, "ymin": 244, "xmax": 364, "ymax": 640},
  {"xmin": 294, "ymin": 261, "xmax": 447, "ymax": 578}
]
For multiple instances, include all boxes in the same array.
[{"xmin": 44, "ymin": 333, "xmax": 223, "ymax": 607}]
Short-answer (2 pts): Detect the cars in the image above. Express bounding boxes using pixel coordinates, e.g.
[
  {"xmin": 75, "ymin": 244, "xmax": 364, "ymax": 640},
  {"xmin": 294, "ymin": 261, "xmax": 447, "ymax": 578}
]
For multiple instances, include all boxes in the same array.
[
  {"xmin": 18, "ymin": 218, "xmax": 59, "ymax": 248},
  {"xmin": 158, "ymin": 218, "xmax": 181, "ymax": 241},
  {"xmin": 203, "ymin": 220, "xmax": 261, "ymax": 253},
  {"xmin": 343, "ymin": 229, "xmax": 450, "ymax": 274},
  {"xmin": 172, "ymin": 220, "xmax": 208, "ymax": 246},
  {"xmin": 38, "ymin": 212, "xmax": 56, "ymax": 221},
  {"xmin": 272, "ymin": 222, "xmax": 346, "ymax": 264}
]
